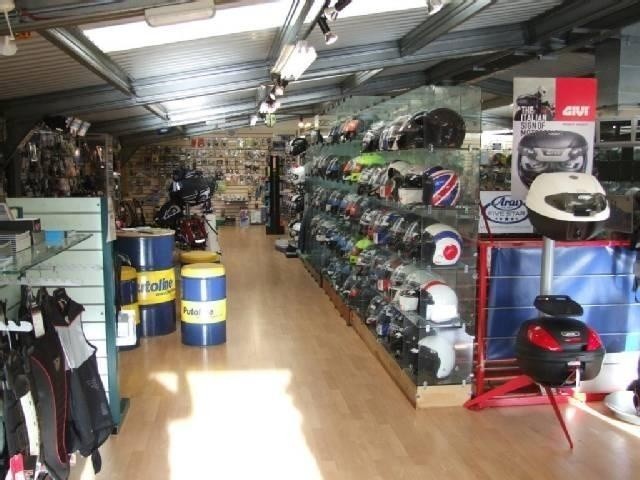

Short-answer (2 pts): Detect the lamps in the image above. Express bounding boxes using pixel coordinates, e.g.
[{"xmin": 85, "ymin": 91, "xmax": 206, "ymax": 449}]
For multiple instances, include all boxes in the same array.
[
  {"xmin": 0, "ymin": 0, "xmax": 19, "ymax": 57},
  {"xmin": 260, "ymin": 81, "xmax": 287, "ymax": 114},
  {"xmin": 144, "ymin": 1, "xmax": 215, "ymax": 27},
  {"xmin": 273, "ymin": 40, "xmax": 318, "ymax": 82},
  {"xmin": 318, "ymin": 0, "xmax": 351, "ymax": 45}
]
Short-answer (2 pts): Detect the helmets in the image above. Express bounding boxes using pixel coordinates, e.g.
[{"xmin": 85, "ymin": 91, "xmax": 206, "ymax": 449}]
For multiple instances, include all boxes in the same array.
[{"xmin": 280, "ymin": 107, "xmax": 465, "ymax": 379}]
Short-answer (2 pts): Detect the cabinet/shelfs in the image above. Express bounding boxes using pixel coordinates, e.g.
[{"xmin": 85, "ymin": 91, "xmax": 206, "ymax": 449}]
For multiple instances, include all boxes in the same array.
[
  {"xmin": 293, "ymin": 85, "xmax": 487, "ymax": 408},
  {"xmin": 0, "ymin": 194, "xmax": 106, "ymax": 480}
]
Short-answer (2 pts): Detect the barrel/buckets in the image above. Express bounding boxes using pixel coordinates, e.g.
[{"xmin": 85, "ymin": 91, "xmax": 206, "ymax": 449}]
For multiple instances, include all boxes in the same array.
[
  {"xmin": 179, "ymin": 251, "xmax": 220, "ymax": 264},
  {"xmin": 116, "ymin": 266, "xmax": 141, "ymax": 349},
  {"xmin": 116, "ymin": 226, "xmax": 176, "ymax": 336},
  {"xmin": 180, "ymin": 263, "xmax": 227, "ymax": 347}
]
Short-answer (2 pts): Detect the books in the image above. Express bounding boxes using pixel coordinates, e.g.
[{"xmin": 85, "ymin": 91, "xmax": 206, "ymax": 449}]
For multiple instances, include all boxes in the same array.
[{"xmin": 1, "ymin": 228, "xmax": 32, "ymax": 252}]
